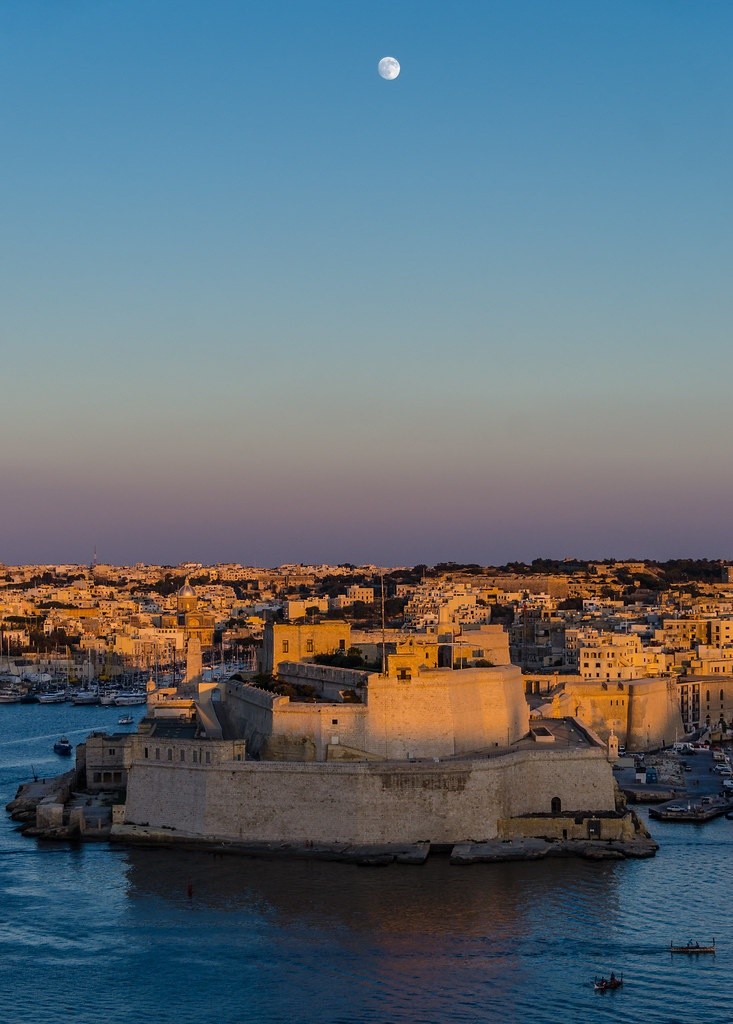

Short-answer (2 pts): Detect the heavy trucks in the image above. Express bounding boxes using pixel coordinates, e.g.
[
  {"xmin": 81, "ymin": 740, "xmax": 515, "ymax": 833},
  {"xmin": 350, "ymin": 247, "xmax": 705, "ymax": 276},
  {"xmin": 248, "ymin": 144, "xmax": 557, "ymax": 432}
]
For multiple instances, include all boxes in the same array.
[{"xmin": 674, "ymin": 742, "xmax": 694, "ymax": 751}]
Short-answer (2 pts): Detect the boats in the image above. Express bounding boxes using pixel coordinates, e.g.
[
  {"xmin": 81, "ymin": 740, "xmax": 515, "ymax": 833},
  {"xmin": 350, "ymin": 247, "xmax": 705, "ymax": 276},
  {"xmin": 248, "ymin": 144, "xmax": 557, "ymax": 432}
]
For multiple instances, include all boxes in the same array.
[
  {"xmin": 0, "ymin": 633, "xmax": 187, "ymax": 706},
  {"xmin": 117, "ymin": 714, "xmax": 134, "ymax": 724},
  {"xmin": 669, "ymin": 938, "xmax": 716, "ymax": 954},
  {"xmin": 593, "ymin": 972, "xmax": 623, "ymax": 990},
  {"xmin": 54, "ymin": 735, "xmax": 74, "ymax": 754}
]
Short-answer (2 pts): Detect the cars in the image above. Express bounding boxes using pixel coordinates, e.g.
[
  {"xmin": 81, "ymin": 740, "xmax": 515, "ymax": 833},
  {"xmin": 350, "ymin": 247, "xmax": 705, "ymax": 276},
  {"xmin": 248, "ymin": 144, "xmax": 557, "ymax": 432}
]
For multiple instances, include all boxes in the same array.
[
  {"xmin": 667, "ymin": 804, "xmax": 685, "ymax": 812},
  {"xmin": 685, "ymin": 766, "xmax": 692, "ymax": 772},
  {"xmin": 711, "ymin": 751, "xmax": 733, "ymax": 791}
]
